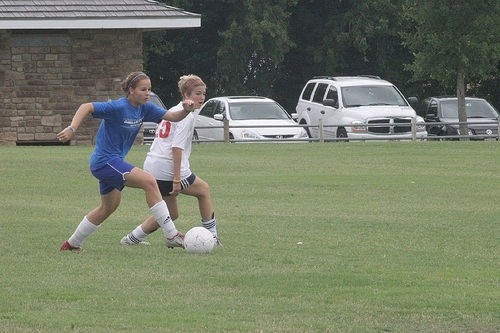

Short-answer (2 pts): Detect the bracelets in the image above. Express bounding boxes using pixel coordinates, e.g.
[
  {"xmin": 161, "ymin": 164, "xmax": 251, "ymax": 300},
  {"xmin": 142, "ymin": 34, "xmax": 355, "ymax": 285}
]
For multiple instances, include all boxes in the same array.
[{"xmin": 172, "ymin": 178, "xmax": 180, "ymax": 183}]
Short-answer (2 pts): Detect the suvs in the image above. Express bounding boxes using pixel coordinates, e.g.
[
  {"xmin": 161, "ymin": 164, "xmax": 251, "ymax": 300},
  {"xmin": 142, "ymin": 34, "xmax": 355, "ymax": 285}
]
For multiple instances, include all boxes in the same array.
[{"xmin": 295, "ymin": 75, "xmax": 429, "ymax": 143}]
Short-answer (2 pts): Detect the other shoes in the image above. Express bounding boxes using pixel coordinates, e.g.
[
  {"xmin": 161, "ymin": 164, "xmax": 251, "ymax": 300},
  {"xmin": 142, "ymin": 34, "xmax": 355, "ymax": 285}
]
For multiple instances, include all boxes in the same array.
[
  {"xmin": 167, "ymin": 230, "xmax": 185, "ymax": 249},
  {"xmin": 211, "ymin": 235, "xmax": 223, "ymax": 247},
  {"xmin": 120, "ymin": 234, "xmax": 151, "ymax": 246},
  {"xmin": 59, "ymin": 241, "xmax": 82, "ymax": 251}
]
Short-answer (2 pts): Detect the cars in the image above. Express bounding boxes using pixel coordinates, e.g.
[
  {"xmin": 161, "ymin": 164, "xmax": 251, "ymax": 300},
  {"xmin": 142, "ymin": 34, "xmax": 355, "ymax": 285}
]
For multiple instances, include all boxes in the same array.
[
  {"xmin": 421, "ymin": 95, "xmax": 500, "ymax": 141},
  {"xmin": 192, "ymin": 95, "xmax": 310, "ymax": 142},
  {"xmin": 143, "ymin": 92, "xmax": 167, "ymax": 140}
]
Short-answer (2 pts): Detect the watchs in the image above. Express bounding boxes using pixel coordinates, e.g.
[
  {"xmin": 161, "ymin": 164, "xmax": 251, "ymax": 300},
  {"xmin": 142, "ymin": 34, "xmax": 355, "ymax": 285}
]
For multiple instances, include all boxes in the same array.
[{"xmin": 69, "ymin": 126, "xmax": 76, "ymax": 133}]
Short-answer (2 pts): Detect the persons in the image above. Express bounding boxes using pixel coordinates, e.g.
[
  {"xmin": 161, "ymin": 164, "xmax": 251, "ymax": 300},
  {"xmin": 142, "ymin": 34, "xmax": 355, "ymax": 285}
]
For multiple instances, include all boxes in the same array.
[
  {"xmin": 121, "ymin": 75, "xmax": 224, "ymax": 248},
  {"xmin": 56, "ymin": 72, "xmax": 194, "ymax": 253}
]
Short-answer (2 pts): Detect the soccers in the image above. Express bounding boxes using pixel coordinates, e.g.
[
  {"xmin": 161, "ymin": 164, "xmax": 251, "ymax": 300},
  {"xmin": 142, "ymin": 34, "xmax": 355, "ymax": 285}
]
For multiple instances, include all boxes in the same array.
[{"xmin": 183, "ymin": 226, "xmax": 214, "ymax": 254}]
